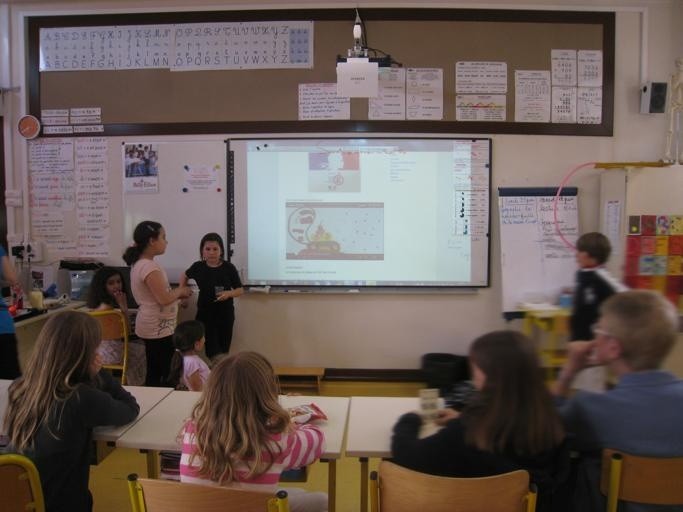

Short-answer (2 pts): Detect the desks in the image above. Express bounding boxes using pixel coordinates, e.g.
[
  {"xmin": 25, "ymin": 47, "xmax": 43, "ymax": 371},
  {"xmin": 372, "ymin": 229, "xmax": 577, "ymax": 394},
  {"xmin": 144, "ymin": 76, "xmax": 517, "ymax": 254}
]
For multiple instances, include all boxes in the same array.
[
  {"xmin": 117, "ymin": 391, "xmax": 352, "ymax": 511},
  {"xmin": 2, "ymin": 378, "xmax": 175, "ymax": 468},
  {"xmin": 344, "ymin": 397, "xmax": 462, "ymax": 511},
  {"xmin": 0, "ymin": 291, "xmax": 139, "ymax": 373}
]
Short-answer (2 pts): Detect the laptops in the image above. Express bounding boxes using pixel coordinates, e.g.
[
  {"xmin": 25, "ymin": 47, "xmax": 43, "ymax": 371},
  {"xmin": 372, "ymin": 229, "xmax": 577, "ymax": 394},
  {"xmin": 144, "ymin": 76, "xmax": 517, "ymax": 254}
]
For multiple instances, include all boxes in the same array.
[{"xmin": 105, "ymin": 266, "xmax": 139, "ymax": 308}]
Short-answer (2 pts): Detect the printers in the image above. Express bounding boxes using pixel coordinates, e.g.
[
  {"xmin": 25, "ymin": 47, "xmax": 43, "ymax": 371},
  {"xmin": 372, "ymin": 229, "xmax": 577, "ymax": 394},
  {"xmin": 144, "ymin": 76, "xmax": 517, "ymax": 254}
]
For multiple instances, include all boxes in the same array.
[{"xmin": 57, "ymin": 257, "xmax": 104, "ymax": 301}]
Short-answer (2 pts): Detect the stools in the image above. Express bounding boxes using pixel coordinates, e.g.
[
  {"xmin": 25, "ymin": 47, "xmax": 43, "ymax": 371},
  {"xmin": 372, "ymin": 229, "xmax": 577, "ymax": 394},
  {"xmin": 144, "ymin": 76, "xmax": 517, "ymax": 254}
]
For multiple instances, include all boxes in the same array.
[{"xmin": 272, "ymin": 367, "xmax": 326, "ymax": 396}]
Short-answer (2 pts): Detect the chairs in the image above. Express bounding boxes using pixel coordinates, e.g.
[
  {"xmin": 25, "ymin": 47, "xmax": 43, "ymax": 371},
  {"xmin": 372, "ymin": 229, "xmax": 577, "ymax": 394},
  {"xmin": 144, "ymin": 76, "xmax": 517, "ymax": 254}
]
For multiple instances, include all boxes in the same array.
[
  {"xmin": 125, "ymin": 472, "xmax": 290, "ymax": 511},
  {"xmin": 600, "ymin": 446, "xmax": 683, "ymax": 511},
  {"xmin": 367, "ymin": 459, "xmax": 538, "ymax": 511},
  {"xmin": 1, "ymin": 452, "xmax": 48, "ymax": 511},
  {"xmin": 69, "ymin": 310, "xmax": 135, "ymax": 389}
]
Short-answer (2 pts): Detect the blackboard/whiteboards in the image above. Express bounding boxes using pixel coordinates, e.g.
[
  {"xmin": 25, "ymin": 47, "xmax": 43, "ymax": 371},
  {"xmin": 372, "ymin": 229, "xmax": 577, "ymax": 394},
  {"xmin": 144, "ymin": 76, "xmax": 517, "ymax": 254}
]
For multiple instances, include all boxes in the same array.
[
  {"xmin": 27, "ymin": 133, "xmax": 479, "ymax": 298},
  {"xmin": 497, "ymin": 187, "xmax": 579, "ymax": 320}
]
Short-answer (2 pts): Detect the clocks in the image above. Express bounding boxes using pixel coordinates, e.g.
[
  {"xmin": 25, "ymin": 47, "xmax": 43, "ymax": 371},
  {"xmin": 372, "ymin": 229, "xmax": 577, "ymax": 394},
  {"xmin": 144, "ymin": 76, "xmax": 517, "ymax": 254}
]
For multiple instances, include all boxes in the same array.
[{"xmin": 17, "ymin": 114, "xmax": 41, "ymax": 139}]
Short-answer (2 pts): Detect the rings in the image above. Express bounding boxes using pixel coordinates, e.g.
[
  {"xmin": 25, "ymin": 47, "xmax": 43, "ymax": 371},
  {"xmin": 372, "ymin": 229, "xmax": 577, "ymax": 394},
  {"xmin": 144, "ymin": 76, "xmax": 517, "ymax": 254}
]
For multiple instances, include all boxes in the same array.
[{"xmin": 223, "ymin": 299, "xmax": 224, "ymax": 301}]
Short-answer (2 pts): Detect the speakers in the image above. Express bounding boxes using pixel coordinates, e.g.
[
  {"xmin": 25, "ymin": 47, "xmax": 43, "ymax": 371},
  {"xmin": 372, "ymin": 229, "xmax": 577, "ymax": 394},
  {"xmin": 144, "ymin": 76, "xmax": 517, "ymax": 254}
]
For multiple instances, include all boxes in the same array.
[{"xmin": 648, "ymin": 80, "xmax": 667, "ymax": 114}]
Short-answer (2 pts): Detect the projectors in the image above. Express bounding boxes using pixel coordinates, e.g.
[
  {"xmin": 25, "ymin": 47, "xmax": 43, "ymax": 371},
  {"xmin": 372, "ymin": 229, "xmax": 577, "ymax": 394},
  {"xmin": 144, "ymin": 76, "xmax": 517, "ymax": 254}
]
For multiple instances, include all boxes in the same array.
[{"xmin": 335, "ymin": 53, "xmax": 402, "ymax": 74}]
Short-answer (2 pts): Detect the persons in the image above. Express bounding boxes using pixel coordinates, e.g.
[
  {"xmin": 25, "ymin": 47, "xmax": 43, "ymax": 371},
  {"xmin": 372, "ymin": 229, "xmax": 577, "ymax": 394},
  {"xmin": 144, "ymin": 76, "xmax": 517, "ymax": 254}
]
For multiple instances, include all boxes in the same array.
[
  {"xmin": 179, "ymin": 232, "xmax": 244, "ymax": 361},
  {"xmin": 3, "ymin": 311, "xmax": 140, "ymax": 512},
  {"xmin": 0, "ymin": 244, "xmax": 22, "ymax": 379},
  {"xmin": 175, "ymin": 351, "xmax": 328, "ymax": 512},
  {"xmin": 390, "ymin": 330, "xmax": 601, "ymax": 512},
  {"xmin": 551, "ymin": 288, "xmax": 683, "ymax": 512},
  {"xmin": 569, "ymin": 232, "xmax": 629, "ymax": 389},
  {"xmin": 87, "ymin": 267, "xmax": 147, "ymax": 386},
  {"xmin": 122, "ymin": 220, "xmax": 193, "ymax": 387},
  {"xmin": 166, "ymin": 320, "xmax": 210, "ymax": 391}
]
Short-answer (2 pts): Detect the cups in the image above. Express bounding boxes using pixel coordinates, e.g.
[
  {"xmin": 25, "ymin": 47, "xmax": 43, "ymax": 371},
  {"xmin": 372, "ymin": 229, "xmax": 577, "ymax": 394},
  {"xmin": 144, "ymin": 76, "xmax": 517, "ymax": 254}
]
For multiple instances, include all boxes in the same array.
[{"xmin": 29, "ymin": 291, "xmax": 42, "ymax": 308}]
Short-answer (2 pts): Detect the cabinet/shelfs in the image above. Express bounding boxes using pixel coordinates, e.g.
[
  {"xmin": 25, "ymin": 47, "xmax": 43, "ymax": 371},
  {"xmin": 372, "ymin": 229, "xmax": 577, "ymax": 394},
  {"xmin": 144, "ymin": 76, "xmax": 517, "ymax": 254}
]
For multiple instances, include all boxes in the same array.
[{"xmin": 595, "ymin": 161, "xmax": 683, "ymax": 382}]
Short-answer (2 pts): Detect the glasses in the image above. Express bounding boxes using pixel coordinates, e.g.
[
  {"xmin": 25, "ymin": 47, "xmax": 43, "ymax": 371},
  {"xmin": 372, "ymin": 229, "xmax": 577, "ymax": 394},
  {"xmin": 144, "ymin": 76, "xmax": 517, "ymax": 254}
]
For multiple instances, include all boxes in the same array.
[{"xmin": 590, "ymin": 323, "xmax": 614, "ymax": 337}]
